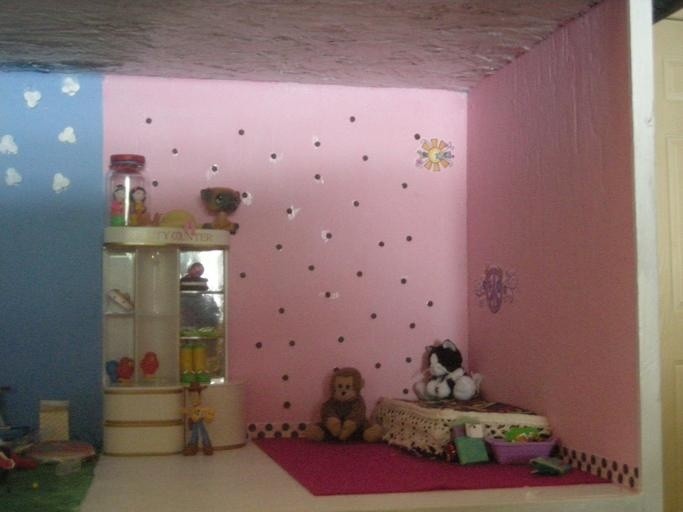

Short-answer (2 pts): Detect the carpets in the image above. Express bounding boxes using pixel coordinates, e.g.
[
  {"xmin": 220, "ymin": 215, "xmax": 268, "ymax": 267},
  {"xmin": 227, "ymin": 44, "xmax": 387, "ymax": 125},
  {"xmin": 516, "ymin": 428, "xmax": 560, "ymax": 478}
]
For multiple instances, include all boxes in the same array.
[
  {"xmin": 252, "ymin": 436, "xmax": 613, "ymax": 496},
  {"xmin": 0, "ymin": 430, "xmax": 102, "ymax": 512}
]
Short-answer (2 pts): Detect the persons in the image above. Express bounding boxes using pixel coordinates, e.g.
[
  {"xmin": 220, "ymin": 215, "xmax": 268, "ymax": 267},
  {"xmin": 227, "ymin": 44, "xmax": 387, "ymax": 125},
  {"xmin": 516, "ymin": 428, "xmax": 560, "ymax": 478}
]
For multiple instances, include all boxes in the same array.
[
  {"xmin": 129, "ymin": 186, "xmax": 161, "ymax": 226},
  {"xmin": 110, "ymin": 184, "xmax": 129, "ymax": 225},
  {"xmin": 176, "ymin": 381, "xmax": 216, "ymax": 455}
]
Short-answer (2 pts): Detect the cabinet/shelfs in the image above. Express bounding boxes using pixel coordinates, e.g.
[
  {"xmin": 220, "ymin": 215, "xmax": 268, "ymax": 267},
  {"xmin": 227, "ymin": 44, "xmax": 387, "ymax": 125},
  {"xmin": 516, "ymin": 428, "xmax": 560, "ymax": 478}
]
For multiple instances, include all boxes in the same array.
[{"xmin": 103, "ymin": 227, "xmax": 247, "ymax": 457}]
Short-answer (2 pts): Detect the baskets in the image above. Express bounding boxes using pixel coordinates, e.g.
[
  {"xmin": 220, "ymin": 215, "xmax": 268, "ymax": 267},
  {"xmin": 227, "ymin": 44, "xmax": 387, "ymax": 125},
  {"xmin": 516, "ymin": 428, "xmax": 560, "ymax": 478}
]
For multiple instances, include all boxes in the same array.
[{"xmin": 484, "ymin": 434, "xmax": 561, "ymax": 465}]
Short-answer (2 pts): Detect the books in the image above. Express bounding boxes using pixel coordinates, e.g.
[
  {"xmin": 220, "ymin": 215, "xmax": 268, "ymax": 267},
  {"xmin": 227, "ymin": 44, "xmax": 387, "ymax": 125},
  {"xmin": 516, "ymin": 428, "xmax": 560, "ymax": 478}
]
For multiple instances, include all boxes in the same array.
[{"xmin": 450, "ymin": 424, "xmax": 488, "ymax": 466}]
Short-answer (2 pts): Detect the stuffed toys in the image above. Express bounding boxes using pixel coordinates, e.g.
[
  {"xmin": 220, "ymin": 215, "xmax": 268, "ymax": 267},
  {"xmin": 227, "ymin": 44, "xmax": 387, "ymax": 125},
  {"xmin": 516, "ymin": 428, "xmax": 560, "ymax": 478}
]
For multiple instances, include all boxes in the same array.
[
  {"xmin": 198, "ymin": 186, "xmax": 240, "ymax": 235},
  {"xmin": 411, "ymin": 338, "xmax": 486, "ymax": 401},
  {"xmin": 316, "ymin": 364, "xmax": 384, "ymax": 443}
]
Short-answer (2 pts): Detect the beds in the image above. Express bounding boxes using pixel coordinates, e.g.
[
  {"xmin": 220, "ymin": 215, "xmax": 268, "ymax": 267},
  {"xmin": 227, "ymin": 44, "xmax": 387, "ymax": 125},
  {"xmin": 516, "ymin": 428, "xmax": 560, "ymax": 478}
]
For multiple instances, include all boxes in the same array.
[{"xmin": 370, "ymin": 397, "xmax": 547, "ymax": 463}]
